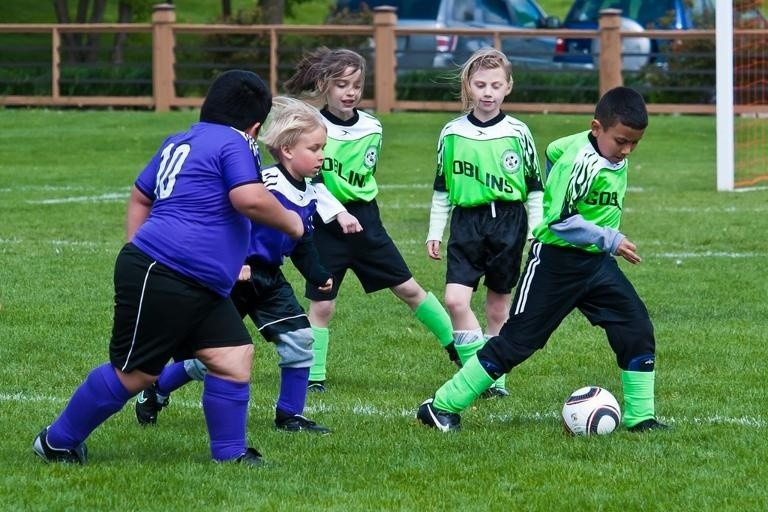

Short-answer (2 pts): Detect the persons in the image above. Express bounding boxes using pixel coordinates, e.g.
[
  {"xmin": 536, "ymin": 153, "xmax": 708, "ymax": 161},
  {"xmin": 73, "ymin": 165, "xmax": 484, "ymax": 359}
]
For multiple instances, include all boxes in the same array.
[
  {"xmin": 30, "ymin": 70, "xmax": 306, "ymax": 468},
  {"xmin": 416, "ymin": 86, "xmax": 668, "ymax": 434},
  {"xmin": 424, "ymin": 46, "xmax": 546, "ymax": 403},
  {"xmin": 136, "ymin": 97, "xmax": 337, "ymax": 438},
  {"xmin": 283, "ymin": 46, "xmax": 463, "ymax": 392}
]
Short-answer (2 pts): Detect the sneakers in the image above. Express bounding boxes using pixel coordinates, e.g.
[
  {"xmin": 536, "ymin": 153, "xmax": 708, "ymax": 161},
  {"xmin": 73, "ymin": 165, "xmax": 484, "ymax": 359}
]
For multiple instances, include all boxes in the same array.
[
  {"xmin": 134, "ymin": 377, "xmax": 170, "ymax": 429},
  {"xmin": 626, "ymin": 418, "xmax": 677, "ymax": 434},
  {"xmin": 415, "ymin": 397, "xmax": 464, "ymax": 436},
  {"xmin": 233, "ymin": 447, "xmax": 275, "ymax": 471},
  {"xmin": 273, "ymin": 412, "xmax": 334, "ymax": 438},
  {"xmin": 30, "ymin": 424, "xmax": 89, "ymax": 470},
  {"xmin": 307, "ymin": 380, "xmax": 327, "ymax": 394},
  {"xmin": 483, "ymin": 386, "xmax": 510, "ymax": 400}
]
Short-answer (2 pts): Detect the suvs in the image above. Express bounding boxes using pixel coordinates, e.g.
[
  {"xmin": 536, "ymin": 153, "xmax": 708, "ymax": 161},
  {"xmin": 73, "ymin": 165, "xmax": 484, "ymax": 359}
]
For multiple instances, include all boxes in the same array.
[
  {"xmin": 366, "ymin": 0, "xmax": 564, "ymax": 79},
  {"xmin": 552, "ymin": 1, "xmax": 767, "ymax": 107}
]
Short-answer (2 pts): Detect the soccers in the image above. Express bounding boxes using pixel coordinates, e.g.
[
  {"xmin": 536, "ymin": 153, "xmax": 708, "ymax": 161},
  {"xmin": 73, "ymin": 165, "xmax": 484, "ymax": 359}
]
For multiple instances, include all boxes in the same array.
[{"xmin": 561, "ymin": 387, "xmax": 621, "ymax": 437}]
